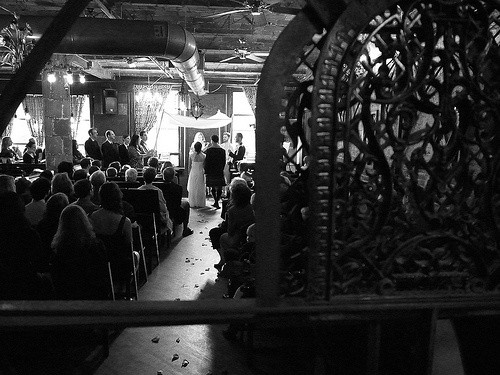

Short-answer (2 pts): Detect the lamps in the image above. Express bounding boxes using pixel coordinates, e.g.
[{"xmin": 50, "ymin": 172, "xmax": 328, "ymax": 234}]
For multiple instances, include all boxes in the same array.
[
  {"xmin": 66, "ymin": 68, "xmax": 74, "ymax": 85},
  {"xmin": 47, "ymin": 68, "xmax": 56, "ymax": 84},
  {"xmin": 78, "ymin": 71, "xmax": 85, "ymax": 84}
]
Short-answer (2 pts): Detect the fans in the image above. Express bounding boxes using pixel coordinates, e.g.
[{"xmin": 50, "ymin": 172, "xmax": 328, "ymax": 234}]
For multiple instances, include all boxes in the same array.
[
  {"xmin": 219, "ymin": 42, "xmax": 266, "ymax": 63},
  {"xmin": 203, "ymin": 0, "xmax": 279, "ymax": 19}
]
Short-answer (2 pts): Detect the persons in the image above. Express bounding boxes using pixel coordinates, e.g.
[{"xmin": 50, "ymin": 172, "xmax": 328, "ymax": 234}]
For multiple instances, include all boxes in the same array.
[
  {"xmin": 84, "ymin": 128, "xmax": 103, "ymax": 160},
  {"xmin": 72, "ymin": 139, "xmax": 86, "ymax": 163},
  {"xmin": 24, "ymin": 137, "xmax": 39, "ymax": 155},
  {"xmin": 228, "ymin": 132, "xmax": 246, "ymax": 167},
  {"xmin": 203, "ymin": 134, "xmax": 228, "ymax": 208},
  {"xmin": 1, "ymin": 157, "xmax": 414, "ymax": 306},
  {"xmin": 139, "ymin": 130, "xmax": 157, "ymax": 156},
  {"xmin": 100, "ymin": 129, "xmax": 121, "ymax": 166},
  {"xmin": 185, "ymin": 141, "xmax": 207, "ymax": 209},
  {"xmin": 220, "ymin": 131, "xmax": 235, "ymax": 197},
  {"xmin": 1, "ymin": 136, "xmax": 19, "ymax": 162},
  {"xmin": 128, "ymin": 134, "xmax": 154, "ymax": 166},
  {"xmin": 23, "ymin": 142, "xmax": 42, "ymax": 163},
  {"xmin": 119, "ymin": 134, "xmax": 131, "ymax": 164},
  {"xmin": 189, "ymin": 131, "xmax": 207, "ymax": 154}
]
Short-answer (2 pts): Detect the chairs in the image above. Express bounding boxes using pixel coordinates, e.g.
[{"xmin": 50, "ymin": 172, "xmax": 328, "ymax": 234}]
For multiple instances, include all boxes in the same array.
[
  {"xmin": 220, "ymin": 166, "xmax": 263, "ymax": 354},
  {"xmin": 0, "ymin": 159, "xmax": 172, "ymax": 375}
]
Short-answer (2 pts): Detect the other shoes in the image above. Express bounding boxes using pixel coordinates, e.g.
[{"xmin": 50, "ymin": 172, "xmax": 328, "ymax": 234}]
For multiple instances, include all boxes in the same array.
[
  {"xmin": 214, "ymin": 264, "xmax": 222, "ymax": 271},
  {"xmin": 223, "ymin": 328, "xmax": 234, "ymax": 342},
  {"xmin": 211, "ymin": 203, "xmax": 217, "ymax": 207},
  {"xmin": 216, "ymin": 205, "xmax": 221, "ymax": 209},
  {"xmin": 223, "ymin": 293, "xmax": 230, "ymax": 298},
  {"xmin": 182, "ymin": 230, "xmax": 193, "ymax": 237}
]
